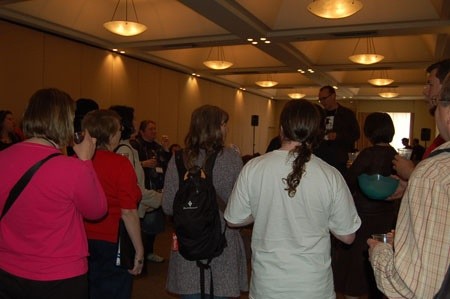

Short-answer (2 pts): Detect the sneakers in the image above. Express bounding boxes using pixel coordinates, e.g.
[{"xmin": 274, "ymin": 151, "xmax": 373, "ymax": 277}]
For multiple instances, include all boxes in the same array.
[{"xmin": 145, "ymin": 253, "xmax": 165, "ymax": 262}]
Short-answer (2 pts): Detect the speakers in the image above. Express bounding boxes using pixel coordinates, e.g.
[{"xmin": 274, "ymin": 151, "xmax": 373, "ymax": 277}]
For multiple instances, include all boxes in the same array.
[
  {"xmin": 251, "ymin": 115, "xmax": 259, "ymax": 126},
  {"xmin": 421, "ymin": 128, "xmax": 431, "ymax": 140}
]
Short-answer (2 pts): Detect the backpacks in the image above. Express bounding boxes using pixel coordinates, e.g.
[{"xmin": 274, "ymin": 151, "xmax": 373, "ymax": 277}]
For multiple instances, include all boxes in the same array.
[{"xmin": 173, "ymin": 145, "xmax": 227, "ymax": 259}]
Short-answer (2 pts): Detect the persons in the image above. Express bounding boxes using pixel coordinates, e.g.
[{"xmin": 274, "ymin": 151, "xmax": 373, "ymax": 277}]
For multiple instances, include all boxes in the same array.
[
  {"xmin": 162, "ymin": 105, "xmax": 247, "ymax": 299},
  {"xmin": 67, "ymin": 98, "xmax": 182, "ymax": 299},
  {"xmin": 402, "ymin": 138, "xmax": 412, "ymax": 149},
  {"xmin": 241, "ymin": 135, "xmax": 282, "ymax": 167},
  {"xmin": 367, "ymin": 73, "xmax": 450, "ymax": 299},
  {"xmin": 0, "ymin": 110, "xmax": 24, "ymax": 151},
  {"xmin": 0, "ymin": 86, "xmax": 108, "ymax": 299},
  {"xmin": 343, "ymin": 112, "xmax": 402, "ymax": 299},
  {"xmin": 385, "ymin": 58, "xmax": 450, "ymax": 200},
  {"xmin": 313, "ymin": 85, "xmax": 360, "ymax": 175},
  {"xmin": 223, "ymin": 98, "xmax": 362, "ymax": 299},
  {"xmin": 409, "ymin": 138, "xmax": 425, "ymax": 165}
]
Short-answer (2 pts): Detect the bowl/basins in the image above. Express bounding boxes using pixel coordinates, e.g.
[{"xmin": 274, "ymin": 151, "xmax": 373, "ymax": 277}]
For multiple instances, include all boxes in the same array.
[{"xmin": 358, "ymin": 174, "xmax": 400, "ymax": 200}]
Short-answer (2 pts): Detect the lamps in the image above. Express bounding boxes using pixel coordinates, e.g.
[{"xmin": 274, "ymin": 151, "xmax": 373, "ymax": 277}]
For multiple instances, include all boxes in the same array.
[
  {"xmin": 203, "ymin": 45, "xmax": 233, "ymax": 70},
  {"xmin": 286, "ymin": 88, "xmax": 307, "ymax": 100},
  {"xmin": 375, "ymin": 89, "xmax": 399, "ymax": 97},
  {"xmin": 253, "ymin": 74, "xmax": 279, "ymax": 88},
  {"xmin": 347, "ymin": 37, "xmax": 385, "ymax": 65},
  {"xmin": 304, "ymin": 0, "xmax": 364, "ymax": 20},
  {"xmin": 366, "ymin": 70, "xmax": 395, "ymax": 86},
  {"xmin": 103, "ymin": 0, "xmax": 148, "ymax": 38}
]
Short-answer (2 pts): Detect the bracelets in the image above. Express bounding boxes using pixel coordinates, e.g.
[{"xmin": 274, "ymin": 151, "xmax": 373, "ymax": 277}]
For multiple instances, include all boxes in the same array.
[
  {"xmin": 135, "ymin": 255, "xmax": 144, "ymax": 264},
  {"xmin": 141, "ymin": 161, "xmax": 144, "ymax": 168}
]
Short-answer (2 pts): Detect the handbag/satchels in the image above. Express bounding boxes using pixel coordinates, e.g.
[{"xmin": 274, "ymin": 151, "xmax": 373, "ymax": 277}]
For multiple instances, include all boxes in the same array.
[{"xmin": 142, "ymin": 206, "xmax": 164, "ymax": 224}]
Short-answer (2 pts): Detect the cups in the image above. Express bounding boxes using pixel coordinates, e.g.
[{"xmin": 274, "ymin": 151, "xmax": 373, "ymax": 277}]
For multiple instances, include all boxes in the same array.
[
  {"xmin": 349, "ymin": 153, "xmax": 356, "ymax": 164},
  {"xmin": 397, "ymin": 148, "xmax": 413, "ymax": 161},
  {"xmin": 372, "ymin": 233, "xmax": 394, "ymax": 250}
]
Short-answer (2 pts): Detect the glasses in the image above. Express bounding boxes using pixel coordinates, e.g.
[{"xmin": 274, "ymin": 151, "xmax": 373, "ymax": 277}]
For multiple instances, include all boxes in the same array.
[
  {"xmin": 317, "ymin": 94, "xmax": 331, "ymax": 104},
  {"xmin": 119, "ymin": 125, "xmax": 125, "ymax": 131},
  {"xmin": 431, "ymin": 95, "xmax": 450, "ymax": 106}
]
